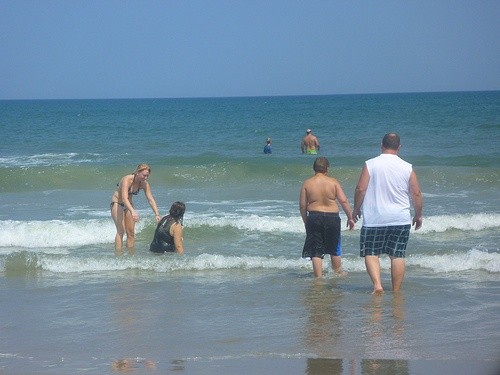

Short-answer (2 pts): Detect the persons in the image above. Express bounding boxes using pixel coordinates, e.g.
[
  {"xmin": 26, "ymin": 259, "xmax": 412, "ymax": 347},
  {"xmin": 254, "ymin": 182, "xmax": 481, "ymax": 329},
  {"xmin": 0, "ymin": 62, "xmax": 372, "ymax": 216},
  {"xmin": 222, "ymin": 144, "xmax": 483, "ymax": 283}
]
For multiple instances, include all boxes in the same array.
[
  {"xmin": 264, "ymin": 138, "xmax": 273, "ymax": 153},
  {"xmin": 300, "ymin": 157, "xmax": 355, "ymax": 277},
  {"xmin": 150, "ymin": 201, "xmax": 186, "ymax": 253},
  {"xmin": 301, "ymin": 128, "xmax": 320, "ymax": 154},
  {"xmin": 110, "ymin": 163, "xmax": 161, "ymax": 249},
  {"xmin": 352, "ymin": 133, "xmax": 423, "ymax": 294}
]
